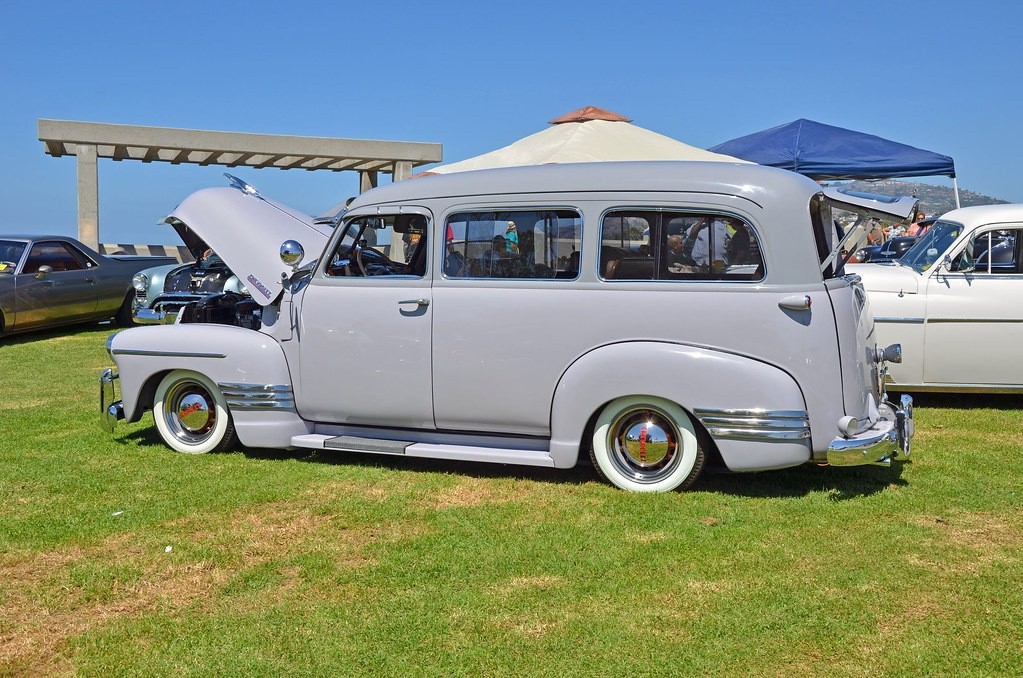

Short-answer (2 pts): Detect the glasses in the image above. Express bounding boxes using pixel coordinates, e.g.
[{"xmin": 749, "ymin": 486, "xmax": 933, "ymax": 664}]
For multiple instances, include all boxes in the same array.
[{"xmin": 917, "ymin": 217, "xmax": 924, "ymax": 220}]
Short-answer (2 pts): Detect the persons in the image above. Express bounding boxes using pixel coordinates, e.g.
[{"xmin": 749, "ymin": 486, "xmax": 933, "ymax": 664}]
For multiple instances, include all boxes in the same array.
[
  {"xmin": 482, "ymin": 220, "xmax": 518, "ymax": 275},
  {"xmin": 866, "ymin": 212, "xmax": 928, "ymax": 245},
  {"xmin": 667, "ymin": 217, "xmax": 750, "ymax": 274},
  {"xmin": 402, "ymin": 224, "xmax": 454, "ymax": 264}
]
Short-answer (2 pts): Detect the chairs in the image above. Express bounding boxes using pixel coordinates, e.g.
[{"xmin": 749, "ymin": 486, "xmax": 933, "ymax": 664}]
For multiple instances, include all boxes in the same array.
[{"xmin": 606, "ymin": 256, "xmax": 656, "ymax": 280}]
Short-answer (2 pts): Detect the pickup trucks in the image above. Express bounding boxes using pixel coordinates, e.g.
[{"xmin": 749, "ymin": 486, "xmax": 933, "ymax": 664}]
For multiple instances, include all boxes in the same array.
[{"xmin": 0, "ymin": 234, "xmax": 180, "ymax": 338}]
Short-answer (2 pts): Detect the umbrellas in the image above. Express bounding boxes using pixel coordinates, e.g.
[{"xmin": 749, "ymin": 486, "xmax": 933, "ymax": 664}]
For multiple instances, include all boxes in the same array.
[{"xmin": 406, "ymin": 105, "xmax": 759, "ymax": 179}]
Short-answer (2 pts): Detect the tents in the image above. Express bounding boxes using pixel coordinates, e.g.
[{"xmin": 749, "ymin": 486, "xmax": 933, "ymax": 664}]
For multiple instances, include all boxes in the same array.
[{"xmin": 707, "ymin": 118, "xmax": 962, "ymax": 261}]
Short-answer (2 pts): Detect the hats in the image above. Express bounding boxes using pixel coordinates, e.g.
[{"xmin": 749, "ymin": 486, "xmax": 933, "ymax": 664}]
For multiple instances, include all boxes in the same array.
[{"xmin": 507, "ymin": 221, "xmax": 516, "ymax": 230}]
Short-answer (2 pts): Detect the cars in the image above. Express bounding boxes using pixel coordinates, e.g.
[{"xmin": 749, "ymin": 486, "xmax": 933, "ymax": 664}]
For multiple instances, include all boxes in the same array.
[
  {"xmin": 131, "ymin": 260, "xmax": 261, "ymax": 331},
  {"xmin": 100, "ymin": 161, "xmax": 920, "ymax": 494},
  {"xmin": 725, "ymin": 203, "xmax": 1023, "ymax": 394}
]
